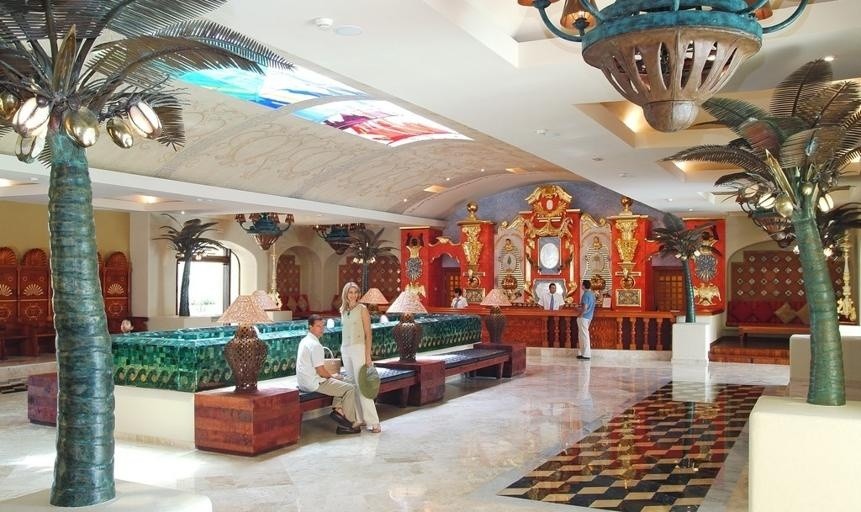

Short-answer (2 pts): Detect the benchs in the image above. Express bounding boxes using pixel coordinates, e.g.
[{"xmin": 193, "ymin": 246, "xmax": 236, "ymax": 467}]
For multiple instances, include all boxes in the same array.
[
  {"xmin": 423, "ymin": 350, "xmax": 510, "ymax": 397},
  {"xmin": 726, "ymin": 300, "xmax": 808, "ymax": 343},
  {"xmin": 300, "ymin": 366, "xmax": 416, "ymax": 433}
]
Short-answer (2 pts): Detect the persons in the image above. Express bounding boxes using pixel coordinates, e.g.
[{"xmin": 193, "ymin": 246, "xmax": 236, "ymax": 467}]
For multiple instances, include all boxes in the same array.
[
  {"xmin": 539, "ymin": 283, "xmax": 562, "ymax": 310},
  {"xmin": 451, "ymin": 288, "xmax": 468, "ymax": 309},
  {"xmin": 296, "ymin": 315, "xmax": 361, "ymax": 434},
  {"xmin": 576, "ymin": 281, "xmax": 598, "ymax": 359},
  {"xmin": 120, "ymin": 318, "xmax": 135, "ymax": 336},
  {"xmin": 338, "ymin": 281, "xmax": 382, "ymax": 433}
]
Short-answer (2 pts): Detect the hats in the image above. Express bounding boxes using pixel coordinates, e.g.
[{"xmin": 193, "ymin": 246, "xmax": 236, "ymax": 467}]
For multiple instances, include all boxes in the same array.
[{"xmin": 357, "ymin": 364, "xmax": 379, "ymax": 400}]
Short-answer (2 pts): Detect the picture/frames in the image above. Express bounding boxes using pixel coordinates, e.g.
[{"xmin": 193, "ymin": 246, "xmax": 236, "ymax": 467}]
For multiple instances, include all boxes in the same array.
[
  {"xmin": 463, "ymin": 288, "xmax": 485, "ymax": 304},
  {"xmin": 616, "ymin": 288, "xmax": 642, "ymax": 306}
]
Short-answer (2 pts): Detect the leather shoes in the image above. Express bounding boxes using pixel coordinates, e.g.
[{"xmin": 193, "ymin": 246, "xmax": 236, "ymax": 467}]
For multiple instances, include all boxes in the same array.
[
  {"xmin": 337, "ymin": 426, "xmax": 361, "ymax": 434},
  {"xmin": 576, "ymin": 355, "xmax": 583, "ymax": 359},
  {"xmin": 330, "ymin": 409, "xmax": 352, "ymax": 428},
  {"xmin": 580, "ymin": 357, "xmax": 590, "ymax": 359}
]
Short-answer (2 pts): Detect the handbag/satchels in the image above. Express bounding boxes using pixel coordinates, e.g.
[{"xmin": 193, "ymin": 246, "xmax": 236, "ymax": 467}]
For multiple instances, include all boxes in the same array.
[{"xmin": 323, "ymin": 358, "xmax": 341, "ymax": 375}]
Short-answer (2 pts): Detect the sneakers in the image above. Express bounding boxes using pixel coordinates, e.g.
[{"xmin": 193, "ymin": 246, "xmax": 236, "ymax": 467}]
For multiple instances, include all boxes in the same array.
[{"xmin": 372, "ymin": 425, "xmax": 381, "ymax": 432}]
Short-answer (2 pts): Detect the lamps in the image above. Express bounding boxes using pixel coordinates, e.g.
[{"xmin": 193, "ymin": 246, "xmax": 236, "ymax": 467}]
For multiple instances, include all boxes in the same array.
[
  {"xmin": 520, "ymin": 1, "xmax": 820, "ymax": 130},
  {"xmin": 314, "ymin": 224, "xmax": 365, "ymax": 256},
  {"xmin": 360, "ymin": 288, "xmax": 389, "ymax": 318},
  {"xmin": 482, "ymin": 289, "xmax": 511, "ymax": 343},
  {"xmin": 741, "ymin": 183, "xmax": 834, "ymax": 214},
  {"xmin": 385, "ymin": 293, "xmax": 428, "ymax": 361},
  {"xmin": 235, "ymin": 214, "xmax": 294, "ymax": 252},
  {"xmin": 253, "ymin": 291, "xmax": 277, "ymax": 312},
  {"xmin": 10, "ymin": 89, "xmax": 164, "ymax": 162},
  {"xmin": 221, "ymin": 295, "xmax": 274, "ymax": 395}
]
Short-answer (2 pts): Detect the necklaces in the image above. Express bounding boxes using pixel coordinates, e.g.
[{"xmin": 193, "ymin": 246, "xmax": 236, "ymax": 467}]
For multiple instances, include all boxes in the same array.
[{"xmin": 348, "ymin": 307, "xmax": 354, "ymax": 316}]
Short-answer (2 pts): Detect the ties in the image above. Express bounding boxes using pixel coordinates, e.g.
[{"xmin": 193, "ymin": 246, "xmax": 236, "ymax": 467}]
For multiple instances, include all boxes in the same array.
[
  {"xmin": 550, "ymin": 294, "xmax": 554, "ymax": 310},
  {"xmin": 453, "ymin": 299, "xmax": 460, "ymax": 308}
]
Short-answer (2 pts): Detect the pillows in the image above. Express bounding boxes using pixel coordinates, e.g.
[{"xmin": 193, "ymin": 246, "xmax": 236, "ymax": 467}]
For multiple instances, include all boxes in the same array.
[
  {"xmin": 287, "ymin": 295, "xmax": 308, "ymax": 313},
  {"xmin": 732, "ymin": 302, "xmax": 811, "ymax": 325}
]
